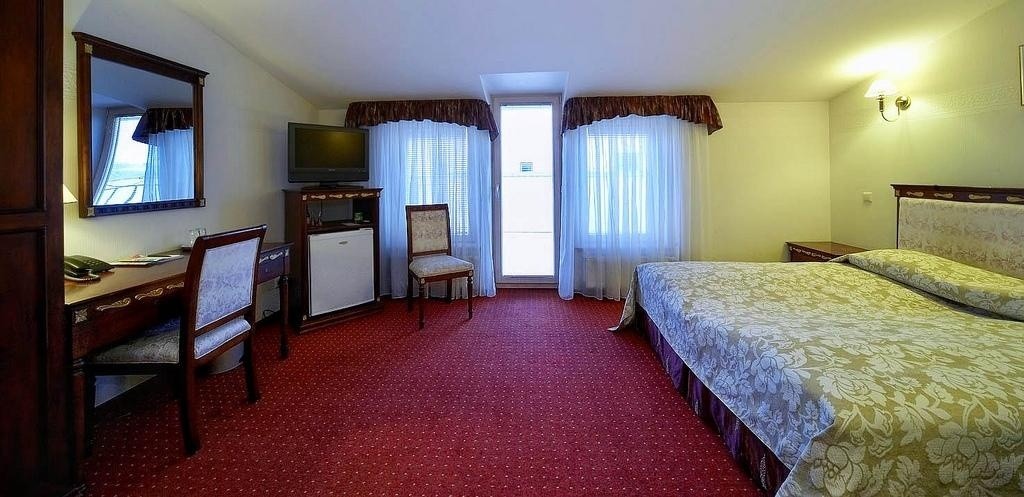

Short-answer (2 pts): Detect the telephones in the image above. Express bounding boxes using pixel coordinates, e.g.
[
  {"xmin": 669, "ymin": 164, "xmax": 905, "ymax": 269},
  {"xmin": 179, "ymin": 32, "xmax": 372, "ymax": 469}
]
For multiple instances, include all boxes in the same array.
[{"xmin": 64, "ymin": 255, "xmax": 115, "ymax": 278}]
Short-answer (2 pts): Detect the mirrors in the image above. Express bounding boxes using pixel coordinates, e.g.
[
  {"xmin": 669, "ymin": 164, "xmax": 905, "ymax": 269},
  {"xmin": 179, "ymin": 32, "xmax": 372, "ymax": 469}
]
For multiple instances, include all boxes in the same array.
[{"xmin": 73, "ymin": 30, "xmax": 210, "ymax": 218}]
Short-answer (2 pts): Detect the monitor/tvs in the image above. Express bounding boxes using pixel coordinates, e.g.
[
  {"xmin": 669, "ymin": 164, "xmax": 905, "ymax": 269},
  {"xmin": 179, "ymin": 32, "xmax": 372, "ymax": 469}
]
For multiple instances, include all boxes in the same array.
[{"xmin": 288, "ymin": 122, "xmax": 370, "ymax": 190}]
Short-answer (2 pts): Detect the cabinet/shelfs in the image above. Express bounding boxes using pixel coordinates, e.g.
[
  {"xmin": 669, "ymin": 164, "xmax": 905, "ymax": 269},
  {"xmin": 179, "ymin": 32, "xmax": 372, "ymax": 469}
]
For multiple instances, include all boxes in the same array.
[{"xmin": 280, "ymin": 188, "xmax": 385, "ymax": 335}]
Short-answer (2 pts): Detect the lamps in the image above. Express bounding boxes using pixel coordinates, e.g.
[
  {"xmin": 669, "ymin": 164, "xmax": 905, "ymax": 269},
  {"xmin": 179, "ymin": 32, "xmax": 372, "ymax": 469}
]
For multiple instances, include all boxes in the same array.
[{"xmin": 865, "ymin": 81, "xmax": 911, "ymax": 121}]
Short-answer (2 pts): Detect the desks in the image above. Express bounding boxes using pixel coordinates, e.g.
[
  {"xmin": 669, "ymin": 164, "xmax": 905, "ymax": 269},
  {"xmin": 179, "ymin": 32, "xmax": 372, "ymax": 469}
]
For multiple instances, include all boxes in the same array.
[{"xmin": 64, "ymin": 241, "xmax": 295, "ymax": 464}]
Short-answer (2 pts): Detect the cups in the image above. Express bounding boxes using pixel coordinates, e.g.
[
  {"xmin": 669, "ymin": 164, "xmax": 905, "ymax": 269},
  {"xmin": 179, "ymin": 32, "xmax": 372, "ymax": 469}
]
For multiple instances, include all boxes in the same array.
[
  {"xmin": 186, "ymin": 226, "xmax": 206, "ymax": 246},
  {"xmin": 354, "ymin": 213, "xmax": 363, "ymax": 224}
]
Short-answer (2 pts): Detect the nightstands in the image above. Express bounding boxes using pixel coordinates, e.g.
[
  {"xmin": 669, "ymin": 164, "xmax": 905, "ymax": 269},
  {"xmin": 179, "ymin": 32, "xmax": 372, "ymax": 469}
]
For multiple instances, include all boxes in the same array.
[{"xmin": 785, "ymin": 241, "xmax": 870, "ymax": 261}]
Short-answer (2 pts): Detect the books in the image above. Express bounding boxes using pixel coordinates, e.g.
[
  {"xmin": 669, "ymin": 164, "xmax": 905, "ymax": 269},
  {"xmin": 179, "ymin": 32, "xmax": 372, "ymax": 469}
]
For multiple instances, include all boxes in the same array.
[{"xmin": 109, "ymin": 254, "xmax": 184, "ymax": 266}]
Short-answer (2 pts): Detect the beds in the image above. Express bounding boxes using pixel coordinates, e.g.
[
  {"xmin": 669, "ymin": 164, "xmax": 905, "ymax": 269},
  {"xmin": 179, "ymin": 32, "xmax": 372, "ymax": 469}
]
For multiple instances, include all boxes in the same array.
[{"xmin": 609, "ymin": 182, "xmax": 1024, "ymax": 497}]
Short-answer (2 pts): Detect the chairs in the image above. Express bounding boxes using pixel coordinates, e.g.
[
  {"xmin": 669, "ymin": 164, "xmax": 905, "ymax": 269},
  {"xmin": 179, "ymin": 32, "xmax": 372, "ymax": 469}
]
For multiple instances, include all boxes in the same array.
[
  {"xmin": 81, "ymin": 224, "xmax": 268, "ymax": 454},
  {"xmin": 407, "ymin": 204, "xmax": 474, "ymax": 329}
]
